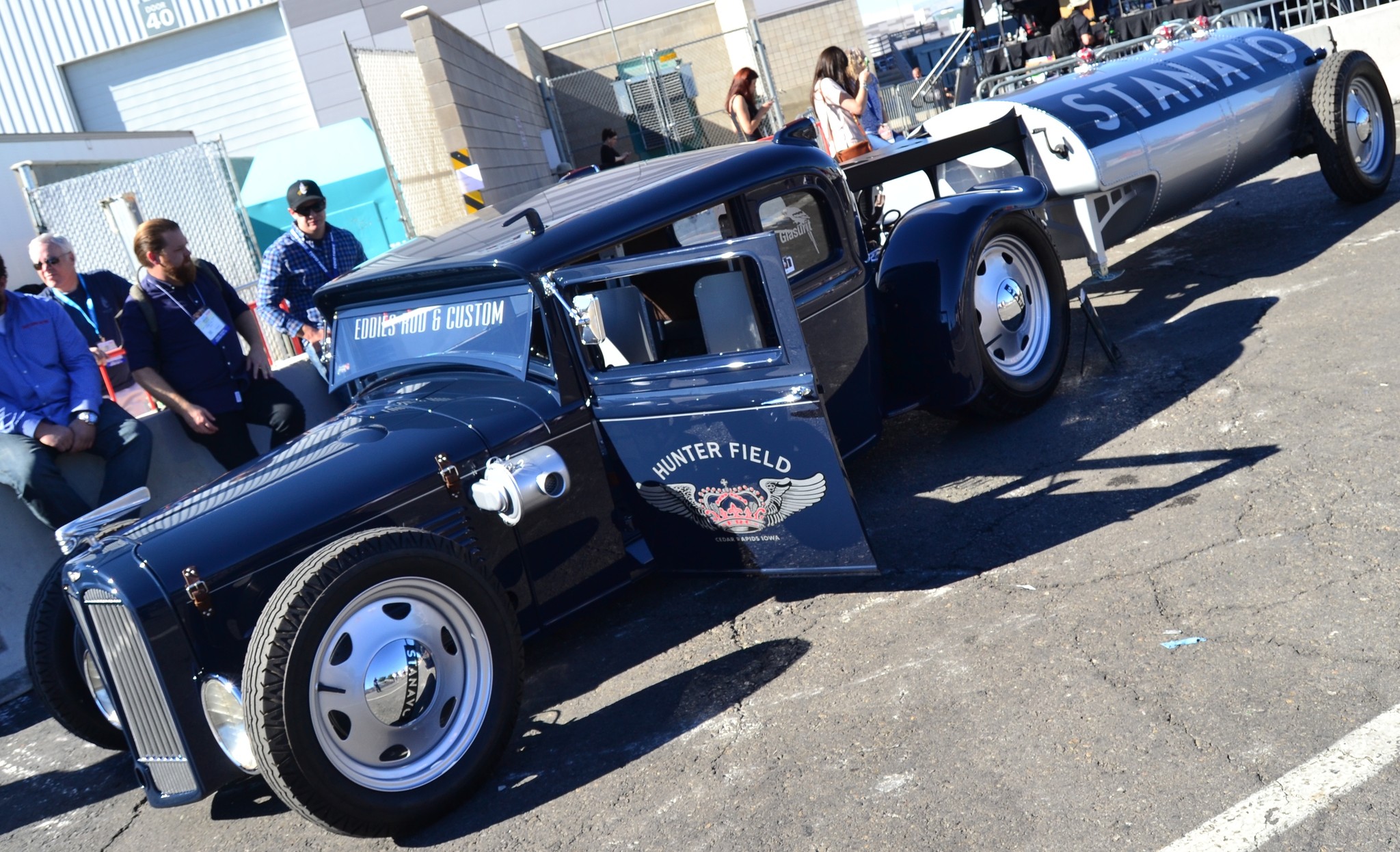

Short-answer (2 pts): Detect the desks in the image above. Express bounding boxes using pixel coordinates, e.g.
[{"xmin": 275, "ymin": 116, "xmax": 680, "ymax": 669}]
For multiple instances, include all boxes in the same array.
[{"xmin": 979, "ymin": 0, "xmax": 1222, "ymax": 77}]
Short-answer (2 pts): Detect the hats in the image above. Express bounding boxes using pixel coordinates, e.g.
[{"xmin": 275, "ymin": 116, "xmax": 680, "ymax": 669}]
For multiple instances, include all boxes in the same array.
[
  {"xmin": 286, "ymin": 179, "xmax": 326, "ymax": 209},
  {"xmin": 1070, "ymin": 0, "xmax": 1089, "ymax": 7}
]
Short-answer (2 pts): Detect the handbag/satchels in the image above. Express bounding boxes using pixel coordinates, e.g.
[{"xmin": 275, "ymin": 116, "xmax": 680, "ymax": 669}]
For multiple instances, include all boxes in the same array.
[{"xmin": 836, "ymin": 140, "xmax": 872, "ymax": 162}]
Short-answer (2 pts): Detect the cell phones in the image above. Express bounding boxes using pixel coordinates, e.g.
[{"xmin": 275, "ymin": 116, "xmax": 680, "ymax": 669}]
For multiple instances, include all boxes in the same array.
[{"xmin": 771, "ymin": 95, "xmax": 774, "ymax": 101}]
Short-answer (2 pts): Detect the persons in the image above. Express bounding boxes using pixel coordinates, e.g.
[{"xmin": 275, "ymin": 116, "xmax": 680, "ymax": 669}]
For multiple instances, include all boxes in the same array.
[
  {"xmin": 843, "ymin": 48, "xmax": 906, "ymax": 150},
  {"xmin": 600, "ymin": 128, "xmax": 631, "ymax": 169},
  {"xmin": 725, "ymin": 67, "xmax": 774, "ymax": 143},
  {"xmin": 1066, "ymin": 0, "xmax": 1098, "ymax": 50},
  {"xmin": 27, "ymin": 233, "xmax": 135, "ymax": 396},
  {"xmin": 0, "ymin": 255, "xmax": 153, "ymax": 531},
  {"xmin": 810, "ymin": 46, "xmax": 875, "ymax": 230},
  {"xmin": 122, "ymin": 219, "xmax": 307, "ymax": 471},
  {"xmin": 257, "ymin": 180, "xmax": 368, "ymax": 383},
  {"xmin": 912, "ymin": 67, "xmax": 955, "ymax": 106}
]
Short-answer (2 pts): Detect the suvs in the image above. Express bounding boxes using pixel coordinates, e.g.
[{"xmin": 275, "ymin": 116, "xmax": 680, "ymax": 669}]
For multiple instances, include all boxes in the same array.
[{"xmin": 24, "ymin": 139, "xmax": 1073, "ymax": 837}]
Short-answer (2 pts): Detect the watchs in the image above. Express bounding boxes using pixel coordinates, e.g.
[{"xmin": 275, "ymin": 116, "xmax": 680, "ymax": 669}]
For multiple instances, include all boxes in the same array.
[{"xmin": 77, "ymin": 412, "xmax": 98, "ymax": 425}]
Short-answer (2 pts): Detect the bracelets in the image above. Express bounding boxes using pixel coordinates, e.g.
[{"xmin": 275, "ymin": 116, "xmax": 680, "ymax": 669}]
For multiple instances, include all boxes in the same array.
[{"xmin": 860, "ymin": 83, "xmax": 869, "ymax": 88}]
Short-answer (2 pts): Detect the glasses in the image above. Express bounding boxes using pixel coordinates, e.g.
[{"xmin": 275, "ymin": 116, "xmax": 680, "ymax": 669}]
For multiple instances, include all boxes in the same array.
[
  {"xmin": 34, "ymin": 253, "xmax": 69, "ymax": 270},
  {"xmin": 294, "ymin": 201, "xmax": 325, "ymax": 215}
]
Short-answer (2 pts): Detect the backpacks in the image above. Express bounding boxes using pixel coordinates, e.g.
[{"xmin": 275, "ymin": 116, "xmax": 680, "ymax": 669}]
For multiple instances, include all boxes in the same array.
[{"xmin": 1051, "ymin": 13, "xmax": 1084, "ymax": 59}]
